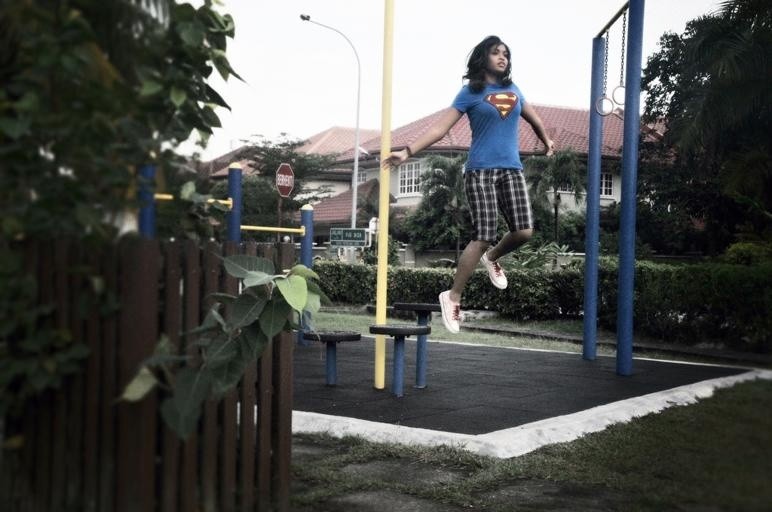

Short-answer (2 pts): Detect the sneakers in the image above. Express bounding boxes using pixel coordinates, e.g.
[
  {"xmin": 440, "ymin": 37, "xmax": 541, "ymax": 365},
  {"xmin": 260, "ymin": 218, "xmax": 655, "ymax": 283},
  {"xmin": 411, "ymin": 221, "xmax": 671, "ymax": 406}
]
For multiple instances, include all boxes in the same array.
[
  {"xmin": 439, "ymin": 290, "xmax": 462, "ymax": 334},
  {"xmin": 480, "ymin": 250, "xmax": 508, "ymax": 290}
]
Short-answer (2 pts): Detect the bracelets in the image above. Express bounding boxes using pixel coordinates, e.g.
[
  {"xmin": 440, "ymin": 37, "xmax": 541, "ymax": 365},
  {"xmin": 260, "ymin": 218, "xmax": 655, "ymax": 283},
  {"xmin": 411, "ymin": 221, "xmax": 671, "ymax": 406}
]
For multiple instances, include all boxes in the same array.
[{"xmin": 405, "ymin": 146, "xmax": 412, "ymax": 157}]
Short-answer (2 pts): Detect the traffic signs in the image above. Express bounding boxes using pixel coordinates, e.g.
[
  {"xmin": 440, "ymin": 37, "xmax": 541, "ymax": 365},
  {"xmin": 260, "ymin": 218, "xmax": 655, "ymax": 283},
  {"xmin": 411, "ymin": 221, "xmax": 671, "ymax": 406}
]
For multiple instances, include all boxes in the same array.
[{"xmin": 329, "ymin": 227, "xmax": 372, "ymax": 249}]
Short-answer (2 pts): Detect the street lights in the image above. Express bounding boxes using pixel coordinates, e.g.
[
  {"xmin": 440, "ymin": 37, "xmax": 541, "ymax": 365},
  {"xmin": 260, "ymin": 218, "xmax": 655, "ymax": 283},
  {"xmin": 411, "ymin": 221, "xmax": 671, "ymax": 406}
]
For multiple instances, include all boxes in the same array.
[{"xmin": 299, "ymin": 12, "xmax": 361, "ymax": 263}]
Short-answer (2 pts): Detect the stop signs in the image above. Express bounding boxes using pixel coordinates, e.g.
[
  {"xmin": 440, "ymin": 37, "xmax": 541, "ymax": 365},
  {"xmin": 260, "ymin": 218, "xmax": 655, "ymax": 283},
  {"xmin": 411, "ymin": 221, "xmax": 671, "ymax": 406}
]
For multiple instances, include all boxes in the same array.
[{"xmin": 276, "ymin": 163, "xmax": 295, "ymax": 198}]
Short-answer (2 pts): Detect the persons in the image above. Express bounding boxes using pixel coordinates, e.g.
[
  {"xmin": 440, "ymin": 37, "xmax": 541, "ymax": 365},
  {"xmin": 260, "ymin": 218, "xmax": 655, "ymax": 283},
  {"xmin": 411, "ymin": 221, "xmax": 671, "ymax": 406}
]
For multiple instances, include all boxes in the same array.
[{"xmin": 380, "ymin": 35, "xmax": 556, "ymax": 334}]
